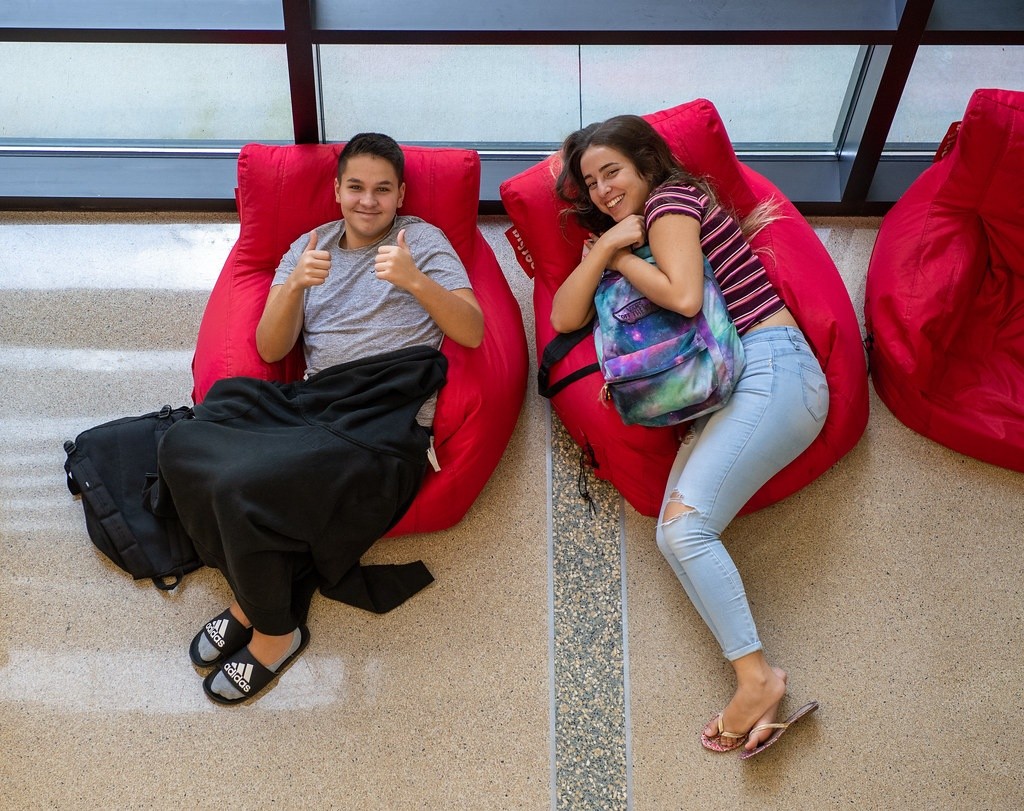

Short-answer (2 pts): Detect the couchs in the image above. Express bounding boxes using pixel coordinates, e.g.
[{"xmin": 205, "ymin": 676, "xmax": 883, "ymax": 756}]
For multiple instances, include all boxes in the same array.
[
  {"xmin": 865, "ymin": 88, "xmax": 1024, "ymax": 473},
  {"xmin": 497, "ymin": 98, "xmax": 869, "ymax": 518},
  {"xmin": 191, "ymin": 143, "xmax": 529, "ymax": 540}
]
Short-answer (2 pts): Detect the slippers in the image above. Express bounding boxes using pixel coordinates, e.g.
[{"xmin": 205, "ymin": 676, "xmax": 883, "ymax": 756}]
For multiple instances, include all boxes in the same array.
[
  {"xmin": 203, "ymin": 621, "xmax": 310, "ymax": 705},
  {"xmin": 740, "ymin": 698, "xmax": 818, "ymax": 760},
  {"xmin": 189, "ymin": 602, "xmax": 257, "ymax": 667},
  {"xmin": 701, "ymin": 706, "xmax": 749, "ymax": 752}
]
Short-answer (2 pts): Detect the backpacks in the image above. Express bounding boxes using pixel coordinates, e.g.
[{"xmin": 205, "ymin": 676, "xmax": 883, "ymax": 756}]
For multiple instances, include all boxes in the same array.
[
  {"xmin": 63, "ymin": 405, "xmax": 207, "ymax": 590},
  {"xmin": 537, "ymin": 246, "xmax": 746, "ymax": 427}
]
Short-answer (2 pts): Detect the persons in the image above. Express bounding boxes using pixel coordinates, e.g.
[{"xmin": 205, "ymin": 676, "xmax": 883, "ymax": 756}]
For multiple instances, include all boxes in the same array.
[
  {"xmin": 549, "ymin": 115, "xmax": 829, "ymax": 759},
  {"xmin": 188, "ymin": 133, "xmax": 484, "ymax": 704}
]
